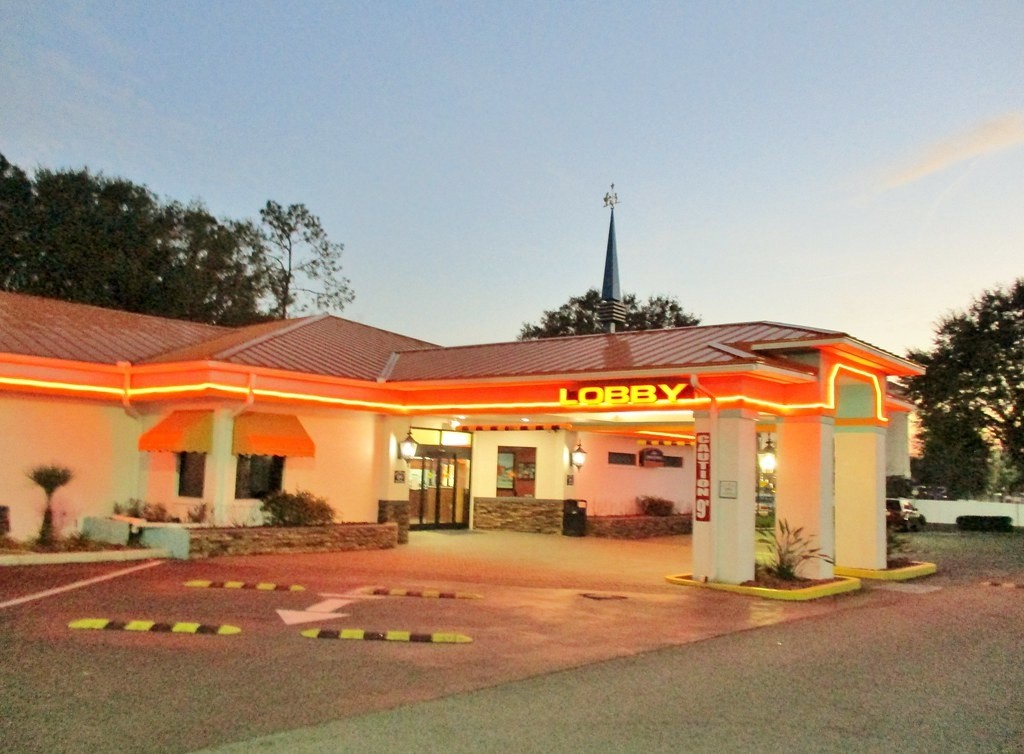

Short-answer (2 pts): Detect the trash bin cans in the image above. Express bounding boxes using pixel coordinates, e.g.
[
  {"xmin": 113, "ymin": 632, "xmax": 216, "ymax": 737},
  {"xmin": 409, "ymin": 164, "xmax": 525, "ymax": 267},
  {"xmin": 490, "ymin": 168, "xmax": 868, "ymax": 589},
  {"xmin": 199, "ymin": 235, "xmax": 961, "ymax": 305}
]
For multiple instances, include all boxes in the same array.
[{"xmin": 562, "ymin": 499, "xmax": 588, "ymax": 537}]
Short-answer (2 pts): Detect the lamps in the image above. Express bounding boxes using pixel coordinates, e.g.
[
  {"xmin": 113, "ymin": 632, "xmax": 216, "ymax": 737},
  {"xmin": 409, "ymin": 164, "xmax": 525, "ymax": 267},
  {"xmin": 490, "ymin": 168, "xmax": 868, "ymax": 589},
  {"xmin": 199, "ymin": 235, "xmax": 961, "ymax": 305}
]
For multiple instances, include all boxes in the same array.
[
  {"xmin": 569, "ymin": 442, "xmax": 587, "ymax": 472},
  {"xmin": 396, "ymin": 428, "xmax": 419, "ymax": 466},
  {"xmin": 756, "ymin": 434, "xmax": 777, "ymax": 483}
]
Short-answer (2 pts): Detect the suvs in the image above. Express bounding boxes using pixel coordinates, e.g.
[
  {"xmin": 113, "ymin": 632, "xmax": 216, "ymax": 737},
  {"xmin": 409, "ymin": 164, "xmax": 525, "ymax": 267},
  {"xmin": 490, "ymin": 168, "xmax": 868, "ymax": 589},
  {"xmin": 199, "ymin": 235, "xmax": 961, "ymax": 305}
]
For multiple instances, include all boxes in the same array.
[{"xmin": 886, "ymin": 498, "xmax": 926, "ymax": 530}]
